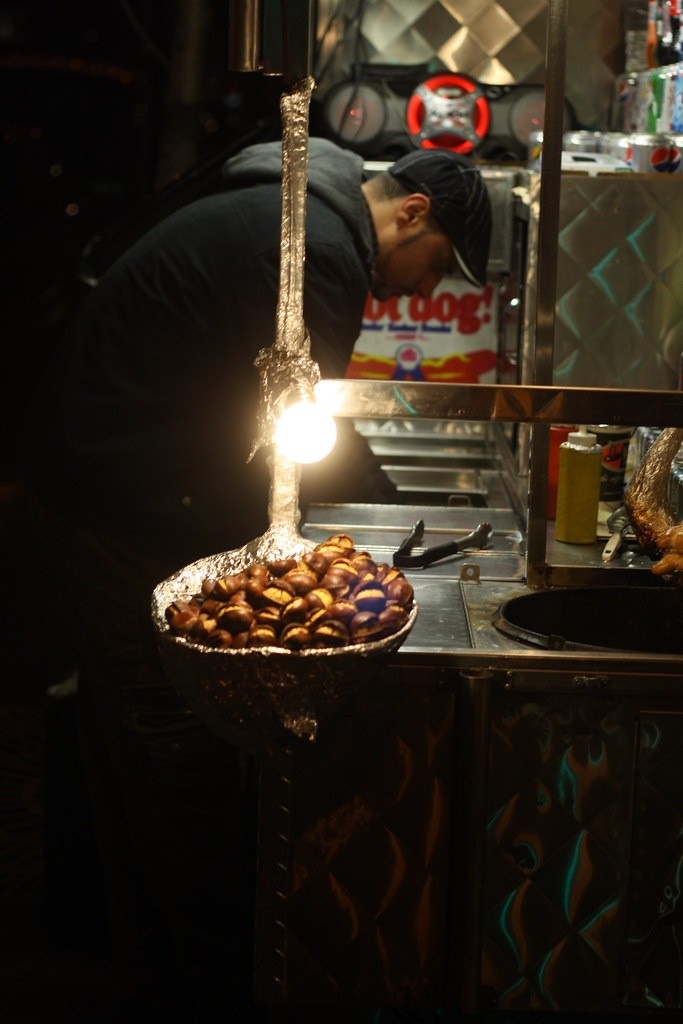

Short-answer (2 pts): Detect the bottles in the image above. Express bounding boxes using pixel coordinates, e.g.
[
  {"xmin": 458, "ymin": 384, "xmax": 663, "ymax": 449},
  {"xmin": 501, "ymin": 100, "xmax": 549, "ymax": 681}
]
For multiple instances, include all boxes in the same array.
[
  {"xmin": 548, "ymin": 424, "xmax": 579, "ymax": 520},
  {"xmin": 554, "ymin": 424, "xmax": 603, "ymax": 545},
  {"xmin": 586, "ymin": 425, "xmax": 632, "ymax": 502},
  {"xmin": 646, "ymin": 0, "xmax": 683, "ymax": 68}
]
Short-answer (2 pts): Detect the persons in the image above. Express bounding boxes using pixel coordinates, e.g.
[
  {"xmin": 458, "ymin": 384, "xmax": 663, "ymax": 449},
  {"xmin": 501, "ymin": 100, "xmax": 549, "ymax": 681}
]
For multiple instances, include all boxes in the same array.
[{"xmin": 0, "ymin": 132, "xmax": 494, "ymax": 1024}]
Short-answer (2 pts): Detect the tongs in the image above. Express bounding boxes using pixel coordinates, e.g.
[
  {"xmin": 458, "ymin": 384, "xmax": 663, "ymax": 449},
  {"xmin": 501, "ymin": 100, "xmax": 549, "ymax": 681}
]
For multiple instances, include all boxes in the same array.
[{"xmin": 392, "ymin": 518, "xmax": 494, "ymax": 568}]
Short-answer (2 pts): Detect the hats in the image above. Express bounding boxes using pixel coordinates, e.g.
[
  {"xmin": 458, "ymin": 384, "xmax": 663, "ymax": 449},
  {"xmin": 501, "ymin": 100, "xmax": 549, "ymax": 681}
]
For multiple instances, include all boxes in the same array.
[{"xmin": 387, "ymin": 148, "xmax": 493, "ymax": 288}]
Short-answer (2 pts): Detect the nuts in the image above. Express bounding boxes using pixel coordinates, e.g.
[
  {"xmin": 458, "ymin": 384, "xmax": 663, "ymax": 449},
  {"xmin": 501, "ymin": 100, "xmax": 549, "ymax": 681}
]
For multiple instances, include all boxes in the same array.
[{"xmin": 165, "ymin": 533, "xmax": 415, "ymax": 650}]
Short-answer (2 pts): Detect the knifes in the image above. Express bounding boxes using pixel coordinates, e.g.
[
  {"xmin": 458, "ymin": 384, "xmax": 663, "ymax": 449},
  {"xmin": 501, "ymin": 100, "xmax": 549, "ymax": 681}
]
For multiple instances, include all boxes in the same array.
[{"xmin": 602, "ymin": 505, "xmax": 631, "ymax": 561}]
